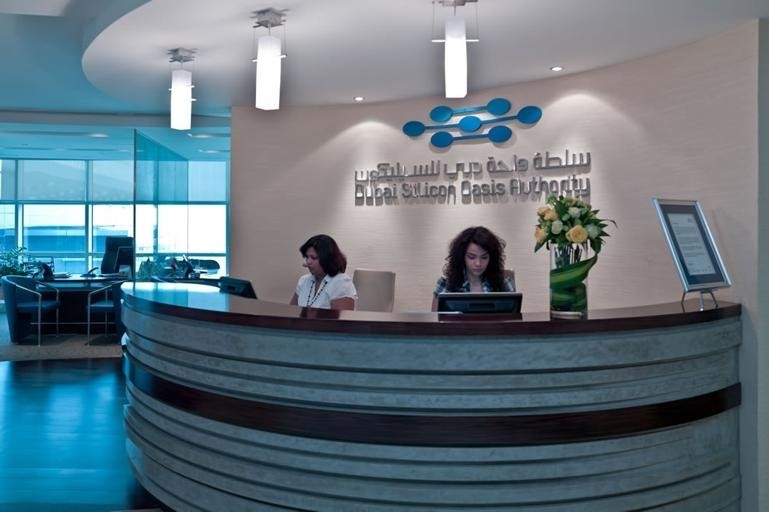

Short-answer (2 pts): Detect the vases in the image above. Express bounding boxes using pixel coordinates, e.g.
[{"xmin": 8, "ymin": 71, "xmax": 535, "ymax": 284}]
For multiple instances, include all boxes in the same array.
[{"xmin": 549, "ymin": 238, "xmax": 588, "ymax": 319}]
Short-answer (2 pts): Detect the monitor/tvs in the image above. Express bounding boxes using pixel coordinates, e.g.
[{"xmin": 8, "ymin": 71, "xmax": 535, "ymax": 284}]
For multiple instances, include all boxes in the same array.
[
  {"xmin": 219, "ymin": 276, "xmax": 258, "ymax": 300},
  {"xmin": 437, "ymin": 290, "xmax": 522, "ymax": 313}
]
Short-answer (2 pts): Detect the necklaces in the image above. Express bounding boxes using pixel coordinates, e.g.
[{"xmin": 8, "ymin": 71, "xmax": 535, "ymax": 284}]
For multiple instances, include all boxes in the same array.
[{"xmin": 306, "ymin": 280, "xmax": 328, "ymax": 306}]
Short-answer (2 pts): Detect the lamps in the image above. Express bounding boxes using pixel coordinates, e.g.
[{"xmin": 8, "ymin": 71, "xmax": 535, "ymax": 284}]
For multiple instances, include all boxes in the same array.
[
  {"xmin": 167, "ymin": 47, "xmax": 195, "ymax": 132},
  {"xmin": 251, "ymin": 8, "xmax": 289, "ymax": 111},
  {"xmin": 433, "ymin": 0, "xmax": 479, "ymax": 99}
]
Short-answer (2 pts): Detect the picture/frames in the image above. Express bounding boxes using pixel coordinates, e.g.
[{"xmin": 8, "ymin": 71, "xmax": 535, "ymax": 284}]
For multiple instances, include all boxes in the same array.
[{"xmin": 653, "ymin": 195, "xmax": 732, "ymax": 294}]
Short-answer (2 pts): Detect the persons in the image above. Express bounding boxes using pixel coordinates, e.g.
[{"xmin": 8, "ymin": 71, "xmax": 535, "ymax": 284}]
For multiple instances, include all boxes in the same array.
[
  {"xmin": 430, "ymin": 224, "xmax": 516, "ymax": 312},
  {"xmin": 287, "ymin": 234, "xmax": 359, "ymax": 312}
]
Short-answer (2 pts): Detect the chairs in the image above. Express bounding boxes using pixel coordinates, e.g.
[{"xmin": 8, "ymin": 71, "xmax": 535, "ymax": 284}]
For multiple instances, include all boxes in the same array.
[
  {"xmin": 1, "ymin": 275, "xmax": 61, "ymax": 346},
  {"xmin": 504, "ymin": 269, "xmax": 515, "ymax": 291},
  {"xmin": 351, "ymin": 269, "xmax": 395, "ymax": 312},
  {"xmin": 85, "ymin": 280, "xmax": 121, "ymax": 346},
  {"xmin": 101, "ymin": 237, "xmax": 132, "ymax": 274}
]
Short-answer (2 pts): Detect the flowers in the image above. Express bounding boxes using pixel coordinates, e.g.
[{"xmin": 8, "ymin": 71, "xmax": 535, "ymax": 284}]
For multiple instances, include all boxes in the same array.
[{"xmin": 533, "ymin": 192, "xmax": 619, "ymax": 271}]
